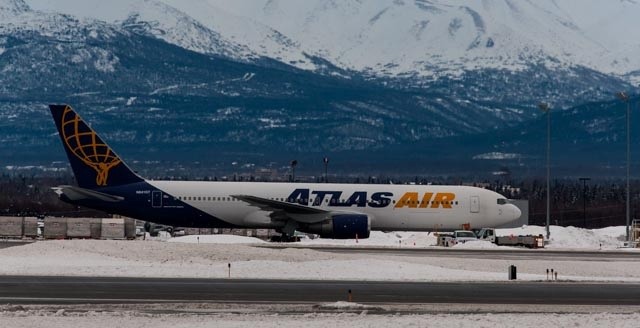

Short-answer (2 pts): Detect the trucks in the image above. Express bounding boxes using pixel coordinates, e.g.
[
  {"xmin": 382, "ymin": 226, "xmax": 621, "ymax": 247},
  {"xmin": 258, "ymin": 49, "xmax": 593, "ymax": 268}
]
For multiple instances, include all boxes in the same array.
[{"xmin": 477, "ymin": 228, "xmax": 536, "ymax": 248}]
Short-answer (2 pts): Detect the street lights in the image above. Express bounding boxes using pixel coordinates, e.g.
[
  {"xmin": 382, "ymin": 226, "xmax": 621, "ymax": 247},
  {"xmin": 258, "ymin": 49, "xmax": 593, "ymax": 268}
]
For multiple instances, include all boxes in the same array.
[
  {"xmin": 616, "ymin": 89, "xmax": 630, "ymax": 242},
  {"xmin": 579, "ymin": 177, "xmax": 591, "ymax": 228},
  {"xmin": 537, "ymin": 103, "xmax": 550, "ymax": 239}
]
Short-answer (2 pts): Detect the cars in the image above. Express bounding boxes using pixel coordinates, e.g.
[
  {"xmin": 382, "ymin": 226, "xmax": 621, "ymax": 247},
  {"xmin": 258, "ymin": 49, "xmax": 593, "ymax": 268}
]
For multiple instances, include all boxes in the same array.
[{"xmin": 454, "ymin": 230, "xmax": 478, "ymax": 244}]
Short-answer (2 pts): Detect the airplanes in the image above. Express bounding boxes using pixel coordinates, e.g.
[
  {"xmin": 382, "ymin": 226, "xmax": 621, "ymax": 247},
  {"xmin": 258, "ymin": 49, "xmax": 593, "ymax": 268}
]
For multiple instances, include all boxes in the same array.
[{"xmin": 49, "ymin": 102, "xmax": 522, "ymax": 242}]
